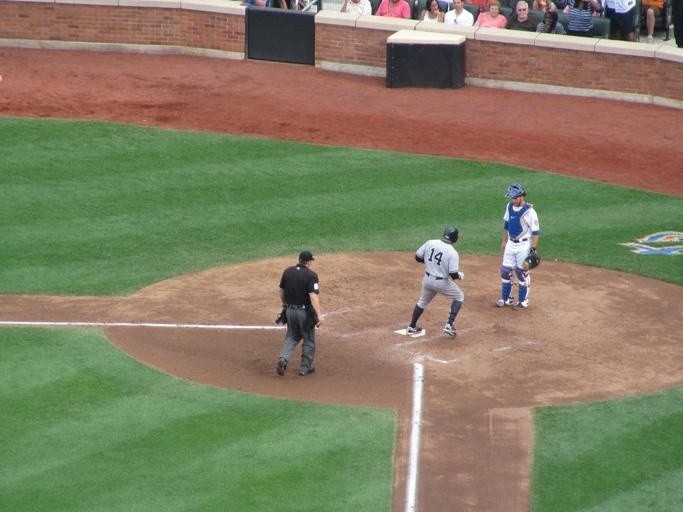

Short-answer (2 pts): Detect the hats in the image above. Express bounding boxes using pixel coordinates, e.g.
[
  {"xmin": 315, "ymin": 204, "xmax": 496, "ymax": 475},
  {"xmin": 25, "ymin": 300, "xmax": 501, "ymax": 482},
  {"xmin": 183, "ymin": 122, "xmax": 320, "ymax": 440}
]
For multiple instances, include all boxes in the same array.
[{"xmin": 298, "ymin": 251, "xmax": 314, "ymax": 261}]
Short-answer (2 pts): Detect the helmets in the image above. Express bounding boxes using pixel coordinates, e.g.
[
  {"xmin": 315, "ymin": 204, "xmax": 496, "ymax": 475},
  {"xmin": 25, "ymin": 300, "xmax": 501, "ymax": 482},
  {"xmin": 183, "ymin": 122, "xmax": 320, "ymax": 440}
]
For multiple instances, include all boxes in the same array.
[
  {"xmin": 505, "ymin": 182, "xmax": 526, "ymax": 199},
  {"xmin": 442, "ymin": 226, "xmax": 458, "ymax": 243}
]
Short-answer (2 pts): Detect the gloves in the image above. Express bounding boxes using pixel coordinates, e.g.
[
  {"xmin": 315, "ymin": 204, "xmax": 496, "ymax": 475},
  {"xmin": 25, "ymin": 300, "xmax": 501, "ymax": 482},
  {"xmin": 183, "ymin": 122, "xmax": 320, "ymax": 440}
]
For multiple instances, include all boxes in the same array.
[{"xmin": 457, "ymin": 272, "xmax": 464, "ymax": 280}]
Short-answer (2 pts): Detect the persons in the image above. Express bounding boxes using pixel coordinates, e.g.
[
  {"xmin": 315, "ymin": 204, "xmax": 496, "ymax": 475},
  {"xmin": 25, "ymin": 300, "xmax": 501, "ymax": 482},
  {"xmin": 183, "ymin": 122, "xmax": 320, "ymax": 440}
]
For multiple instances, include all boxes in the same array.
[
  {"xmin": 496, "ymin": 184, "xmax": 540, "ymax": 311},
  {"xmin": 276, "ymin": 251, "xmax": 323, "ymax": 377},
  {"xmin": 242, "ymin": 1, "xmax": 683, "ymax": 48},
  {"xmin": 407, "ymin": 226, "xmax": 465, "ymax": 336}
]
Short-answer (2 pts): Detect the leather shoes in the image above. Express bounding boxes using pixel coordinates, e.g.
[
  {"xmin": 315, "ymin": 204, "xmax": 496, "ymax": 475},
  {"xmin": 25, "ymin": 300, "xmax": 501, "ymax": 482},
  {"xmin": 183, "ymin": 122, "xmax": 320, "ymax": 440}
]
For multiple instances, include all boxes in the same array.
[
  {"xmin": 277, "ymin": 358, "xmax": 287, "ymax": 376},
  {"xmin": 298, "ymin": 366, "xmax": 315, "ymax": 376}
]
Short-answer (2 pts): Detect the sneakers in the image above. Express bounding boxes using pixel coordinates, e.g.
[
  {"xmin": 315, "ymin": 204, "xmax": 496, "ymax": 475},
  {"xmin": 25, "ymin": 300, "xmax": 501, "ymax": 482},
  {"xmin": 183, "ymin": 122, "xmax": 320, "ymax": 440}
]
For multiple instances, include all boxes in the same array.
[
  {"xmin": 406, "ymin": 326, "xmax": 422, "ymax": 335},
  {"xmin": 444, "ymin": 323, "xmax": 456, "ymax": 336},
  {"xmin": 496, "ymin": 297, "xmax": 513, "ymax": 307},
  {"xmin": 512, "ymin": 299, "xmax": 528, "ymax": 310}
]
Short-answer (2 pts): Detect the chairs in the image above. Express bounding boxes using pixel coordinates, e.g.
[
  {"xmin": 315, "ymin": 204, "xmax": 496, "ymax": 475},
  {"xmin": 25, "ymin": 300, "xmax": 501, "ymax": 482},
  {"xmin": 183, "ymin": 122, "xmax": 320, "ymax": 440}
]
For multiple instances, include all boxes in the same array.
[{"xmin": 368, "ymin": 0, "xmax": 683, "ymax": 56}]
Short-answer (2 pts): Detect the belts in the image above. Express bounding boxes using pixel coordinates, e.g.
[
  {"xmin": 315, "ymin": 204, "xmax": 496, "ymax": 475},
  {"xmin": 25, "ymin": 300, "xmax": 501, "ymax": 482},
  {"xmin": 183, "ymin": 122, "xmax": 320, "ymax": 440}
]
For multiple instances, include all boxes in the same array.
[
  {"xmin": 425, "ymin": 271, "xmax": 445, "ymax": 280},
  {"xmin": 510, "ymin": 238, "xmax": 528, "ymax": 243},
  {"xmin": 286, "ymin": 304, "xmax": 306, "ymax": 309}
]
